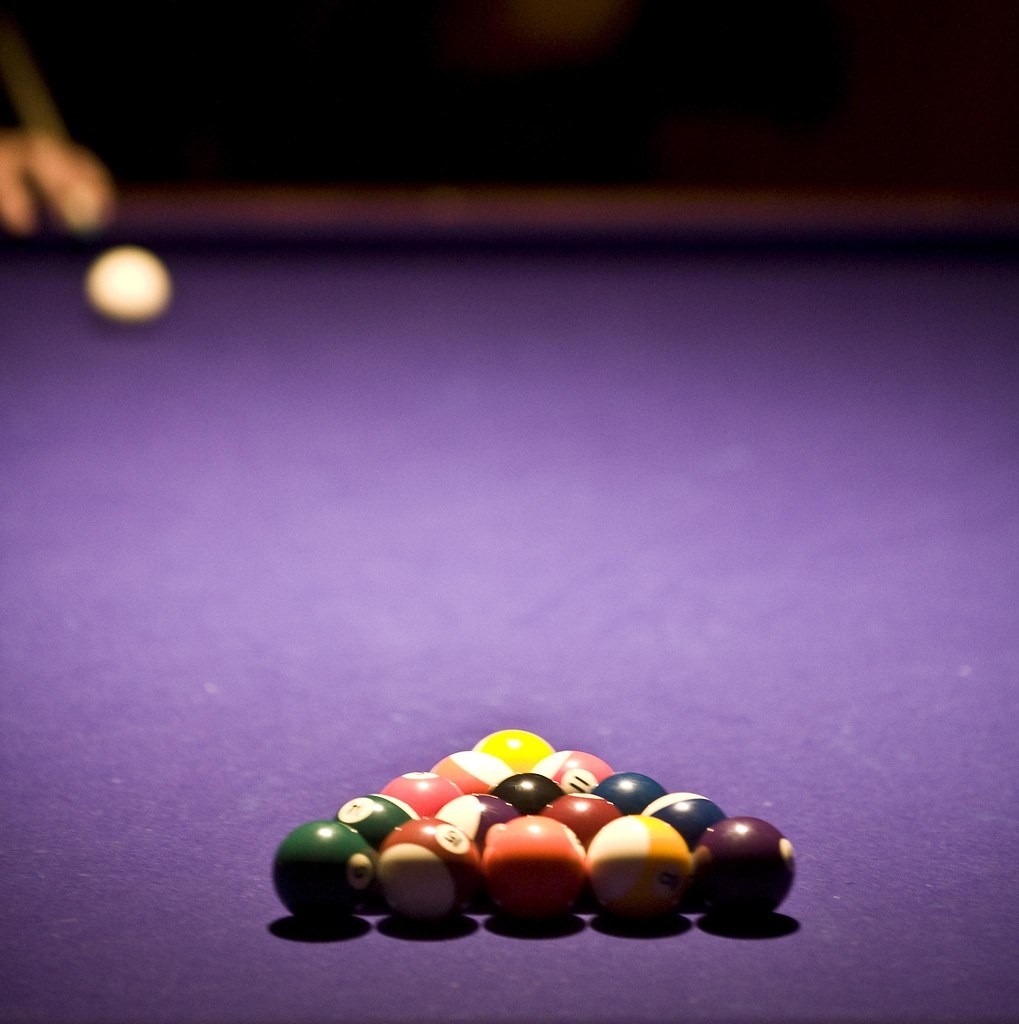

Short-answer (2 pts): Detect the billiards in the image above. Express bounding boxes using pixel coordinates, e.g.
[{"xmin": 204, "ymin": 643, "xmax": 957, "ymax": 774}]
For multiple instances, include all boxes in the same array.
[
  {"xmin": 83, "ymin": 245, "xmax": 172, "ymax": 323},
  {"xmin": 269, "ymin": 728, "xmax": 797, "ymax": 921}
]
